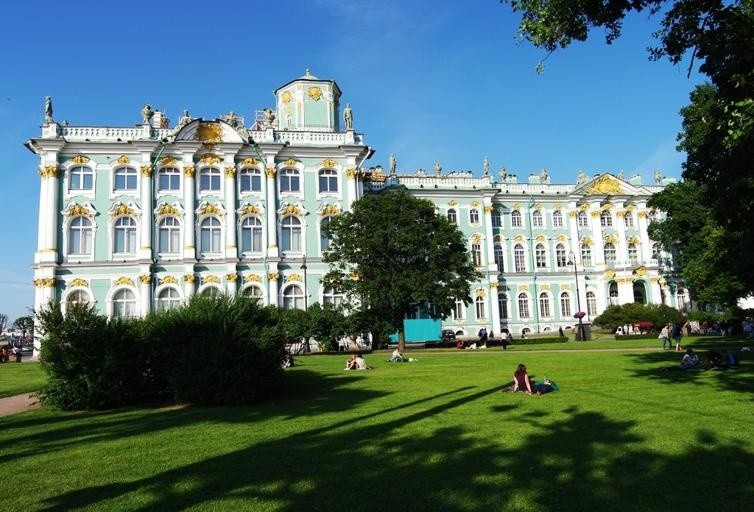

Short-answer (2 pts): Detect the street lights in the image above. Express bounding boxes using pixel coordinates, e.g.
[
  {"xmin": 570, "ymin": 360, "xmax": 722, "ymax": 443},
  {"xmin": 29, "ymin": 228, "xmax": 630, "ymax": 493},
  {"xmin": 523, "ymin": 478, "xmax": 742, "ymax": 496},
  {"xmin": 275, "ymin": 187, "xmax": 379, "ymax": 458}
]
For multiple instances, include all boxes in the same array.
[
  {"xmin": 572, "ymin": 256, "xmax": 587, "ymax": 342},
  {"xmin": 300, "ymin": 254, "xmax": 312, "ymax": 355}
]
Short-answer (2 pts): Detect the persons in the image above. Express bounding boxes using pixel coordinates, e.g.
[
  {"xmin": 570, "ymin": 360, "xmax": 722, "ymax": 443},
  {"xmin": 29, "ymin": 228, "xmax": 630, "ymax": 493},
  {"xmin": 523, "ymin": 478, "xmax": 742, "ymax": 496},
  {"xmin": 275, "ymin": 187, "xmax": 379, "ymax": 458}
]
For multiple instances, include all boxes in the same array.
[
  {"xmin": 343, "ymin": 103, "xmax": 353, "ymax": 129},
  {"xmin": 0, "ymin": 345, "xmax": 22, "ymax": 364},
  {"xmin": 623, "ymin": 315, "xmax": 754, "ymax": 371},
  {"xmin": 394, "ymin": 356, "xmax": 419, "ymax": 362},
  {"xmin": 386, "ymin": 348, "xmax": 402, "ymax": 362},
  {"xmin": 533, "ymin": 379, "xmax": 558, "ymax": 396},
  {"xmin": 280, "ymin": 354, "xmax": 294, "ymax": 368},
  {"xmin": 522, "ymin": 329, "xmax": 525, "ymax": 338},
  {"xmin": 389, "ymin": 153, "xmax": 396, "ymax": 173},
  {"xmin": 511, "ymin": 364, "xmax": 536, "ymax": 395},
  {"xmin": 344, "ymin": 353, "xmax": 372, "ymax": 371},
  {"xmin": 456, "ymin": 328, "xmax": 488, "ymax": 350},
  {"xmin": 499, "ymin": 329, "xmax": 509, "ymax": 349},
  {"xmin": 44, "ymin": 96, "xmax": 53, "ymax": 117}
]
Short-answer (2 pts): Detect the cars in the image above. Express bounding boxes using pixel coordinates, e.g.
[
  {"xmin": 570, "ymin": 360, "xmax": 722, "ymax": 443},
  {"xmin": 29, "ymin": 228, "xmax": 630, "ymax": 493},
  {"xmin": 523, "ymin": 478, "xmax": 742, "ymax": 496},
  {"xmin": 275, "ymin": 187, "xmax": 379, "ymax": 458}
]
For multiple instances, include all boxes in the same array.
[
  {"xmin": 442, "ymin": 329, "xmax": 457, "ymax": 341},
  {"xmin": 0, "ymin": 334, "xmax": 34, "ymax": 353}
]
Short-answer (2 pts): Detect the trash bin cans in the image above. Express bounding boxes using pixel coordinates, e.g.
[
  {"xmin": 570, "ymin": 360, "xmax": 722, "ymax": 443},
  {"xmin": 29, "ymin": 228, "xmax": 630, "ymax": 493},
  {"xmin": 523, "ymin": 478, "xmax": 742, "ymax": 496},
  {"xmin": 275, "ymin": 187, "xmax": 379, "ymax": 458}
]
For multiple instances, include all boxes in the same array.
[
  {"xmin": 384, "ymin": 345, "xmax": 388, "ymax": 349},
  {"xmin": 340, "ymin": 346, "xmax": 344, "ymax": 351},
  {"xmin": 583, "ymin": 323, "xmax": 592, "ymax": 341}
]
